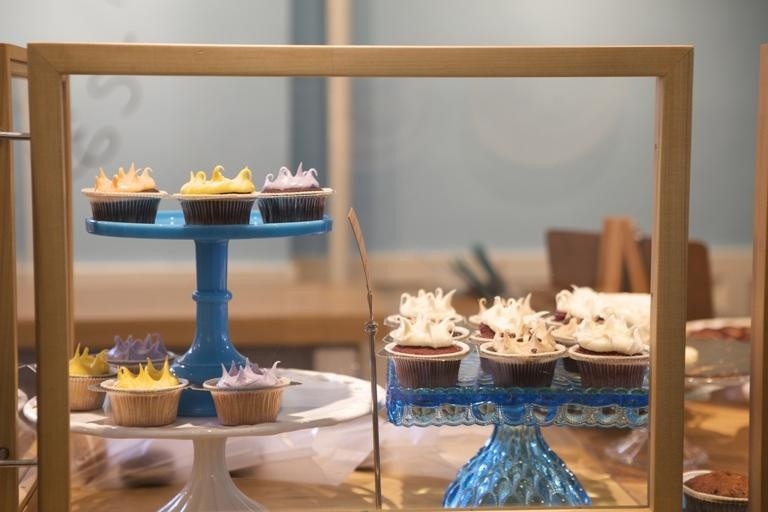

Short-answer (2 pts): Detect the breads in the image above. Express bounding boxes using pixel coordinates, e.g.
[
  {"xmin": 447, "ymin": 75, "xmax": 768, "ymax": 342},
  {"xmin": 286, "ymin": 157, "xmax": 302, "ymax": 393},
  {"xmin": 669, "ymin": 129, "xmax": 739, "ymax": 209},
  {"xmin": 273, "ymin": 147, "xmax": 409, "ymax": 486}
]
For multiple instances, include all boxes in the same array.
[{"xmin": 683, "ymin": 471, "xmax": 749, "ymax": 512}]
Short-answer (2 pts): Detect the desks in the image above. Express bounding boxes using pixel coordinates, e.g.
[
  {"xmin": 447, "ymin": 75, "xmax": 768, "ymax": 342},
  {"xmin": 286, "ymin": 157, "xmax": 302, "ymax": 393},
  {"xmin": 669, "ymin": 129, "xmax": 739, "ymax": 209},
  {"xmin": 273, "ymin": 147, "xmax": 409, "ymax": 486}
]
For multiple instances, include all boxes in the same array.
[{"xmin": 15, "ymin": 248, "xmax": 751, "ymax": 512}]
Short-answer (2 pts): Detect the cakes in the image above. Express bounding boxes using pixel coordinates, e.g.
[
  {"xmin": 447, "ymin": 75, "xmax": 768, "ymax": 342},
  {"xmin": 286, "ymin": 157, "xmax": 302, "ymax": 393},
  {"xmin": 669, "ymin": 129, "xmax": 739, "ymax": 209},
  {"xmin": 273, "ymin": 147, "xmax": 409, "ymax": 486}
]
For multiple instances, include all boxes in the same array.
[
  {"xmin": 98, "ymin": 355, "xmax": 188, "ymax": 428},
  {"xmin": 258, "ymin": 161, "xmax": 334, "ymax": 223},
  {"xmin": 107, "ymin": 332, "xmax": 176, "ymax": 374},
  {"xmin": 81, "ymin": 164, "xmax": 168, "ymax": 223},
  {"xmin": 379, "ymin": 283, "xmax": 651, "ymax": 389},
  {"xmin": 171, "ymin": 165, "xmax": 258, "ymax": 226},
  {"xmin": 202, "ymin": 355, "xmax": 293, "ymax": 426},
  {"xmin": 67, "ymin": 343, "xmax": 118, "ymax": 412}
]
